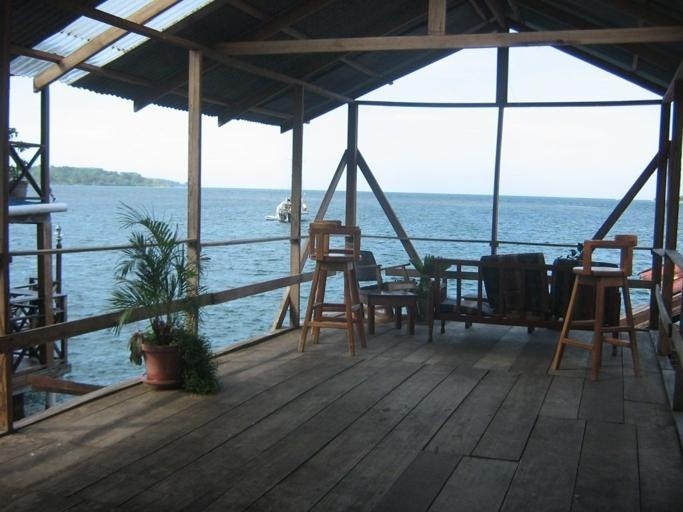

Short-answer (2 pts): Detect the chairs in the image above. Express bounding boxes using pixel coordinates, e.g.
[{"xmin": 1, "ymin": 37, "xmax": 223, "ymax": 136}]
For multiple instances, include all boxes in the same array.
[
  {"xmin": 545, "ymin": 234, "xmax": 641, "ymax": 384},
  {"xmin": 355, "ymin": 251, "xmax": 417, "ymax": 318},
  {"xmin": 296, "ymin": 219, "xmax": 366, "ymax": 357}
]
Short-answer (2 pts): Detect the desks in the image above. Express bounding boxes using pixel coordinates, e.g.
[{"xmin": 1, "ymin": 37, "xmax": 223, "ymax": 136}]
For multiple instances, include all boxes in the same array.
[{"xmin": 364, "ymin": 290, "xmax": 418, "ymax": 335}]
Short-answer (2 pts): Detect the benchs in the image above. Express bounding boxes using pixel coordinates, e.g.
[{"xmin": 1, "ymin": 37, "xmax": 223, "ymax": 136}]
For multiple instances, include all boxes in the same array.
[{"xmin": 385, "ymin": 258, "xmax": 622, "ymax": 357}]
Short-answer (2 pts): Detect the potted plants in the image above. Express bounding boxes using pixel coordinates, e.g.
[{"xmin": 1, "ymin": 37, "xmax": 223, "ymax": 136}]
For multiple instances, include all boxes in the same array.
[{"xmin": 103, "ymin": 198, "xmax": 225, "ymax": 397}]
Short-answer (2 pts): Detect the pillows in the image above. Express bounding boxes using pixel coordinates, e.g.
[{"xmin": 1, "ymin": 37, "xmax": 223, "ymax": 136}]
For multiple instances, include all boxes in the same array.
[{"xmin": 479, "ymin": 252, "xmax": 619, "ymax": 322}]
